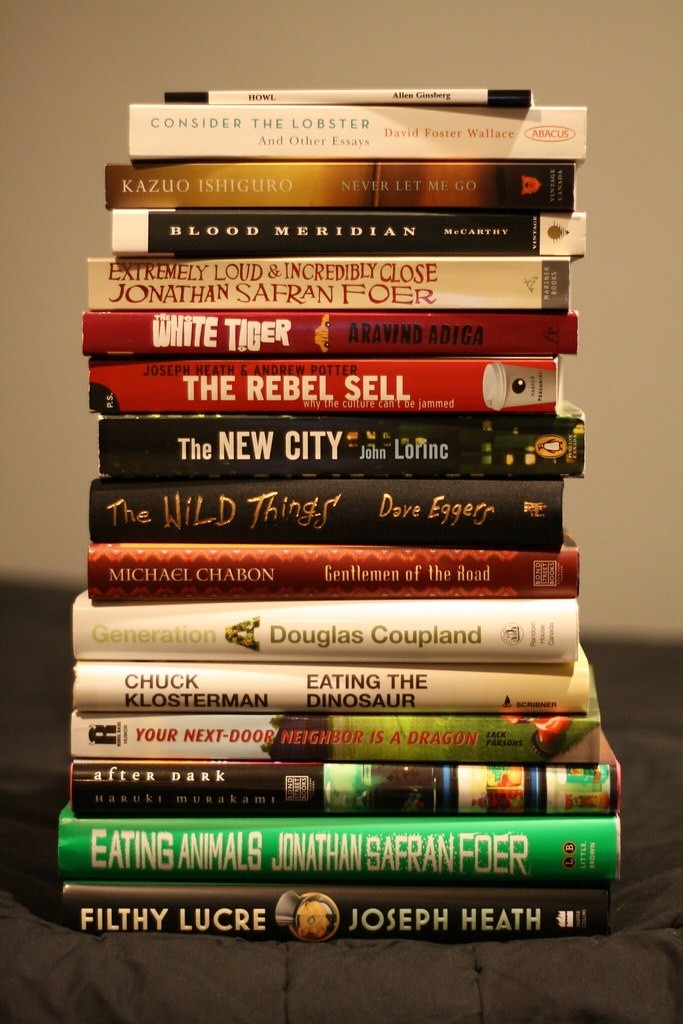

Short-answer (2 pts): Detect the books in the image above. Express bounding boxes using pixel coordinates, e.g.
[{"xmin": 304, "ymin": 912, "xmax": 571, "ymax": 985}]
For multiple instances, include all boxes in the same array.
[
  {"xmin": 164, "ymin": 88, "xmax": 534, "ymax": 110},
  {"xmin": 62, "ymin": 109, "xmax": 624, "ymax": 939}
]
